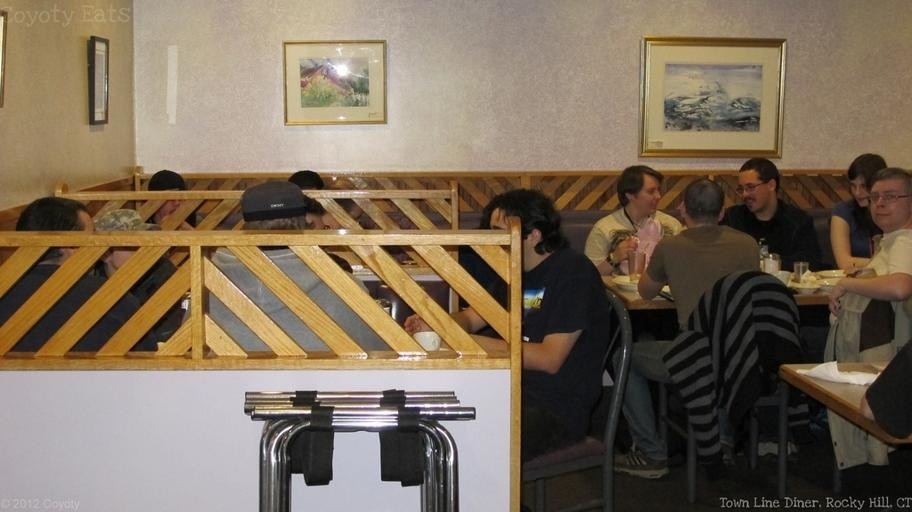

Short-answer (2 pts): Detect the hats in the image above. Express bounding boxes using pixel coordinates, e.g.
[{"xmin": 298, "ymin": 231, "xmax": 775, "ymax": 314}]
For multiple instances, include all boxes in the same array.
[
  {"xmin": 92, "ymin": 209, "xmax": 160, "ymax": 230},
  {"xmin": 241, "ymin": 182, "xmax": 307, "ymax": 219}
]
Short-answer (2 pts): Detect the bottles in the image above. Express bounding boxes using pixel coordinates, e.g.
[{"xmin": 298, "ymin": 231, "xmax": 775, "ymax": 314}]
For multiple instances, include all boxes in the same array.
[{"xmin": 758, "ymin": 238, "xmax": 769, "ymax": 270}]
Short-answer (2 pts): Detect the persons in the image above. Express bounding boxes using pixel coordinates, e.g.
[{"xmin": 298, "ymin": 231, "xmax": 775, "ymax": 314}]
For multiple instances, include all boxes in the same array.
[
  {"xmin": 583, "ymin": 164, "xmax": 684, "ymax": 459},
  {"xmin": 805, "ymin": 164, "xmax": 912, "ymax": 489},
  {"xmin": 401, "ymin": 186, "xmax": 610, "ymax": 512},
  {"xmin": 842, "ymin": 336, "xmax": 911, "ymax": 512},
  {"xmin": 612, "ymin": 179, "xmax": 759, "ymax": 480},
  {"xmin": 718, "ymin": 157, "xmax": 821, "ymax": 448},
  {"xmin": 828, "ymin": 152, "xmax": 886, "ymax": 271}
]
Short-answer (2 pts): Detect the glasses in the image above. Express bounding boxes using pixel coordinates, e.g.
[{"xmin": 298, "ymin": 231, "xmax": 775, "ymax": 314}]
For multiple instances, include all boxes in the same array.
[
  {"xmin": 735, "ymin": 183, "xmax": 762, "ymax": 197},
  {"xmin": 868, "ymin": 195, "xmax": 907, "ymax": 203}
]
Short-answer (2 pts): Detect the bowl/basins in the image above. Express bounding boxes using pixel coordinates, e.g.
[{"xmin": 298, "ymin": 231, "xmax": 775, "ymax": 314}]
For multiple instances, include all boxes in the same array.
[
  {"xmin": 817, "ymin": 268, "xmax": 847, "ymax": 277},
  {"xmin": 818, "ymin": 277, "xmax": 847, "ymax": 292},
  {"xmin": 790, "ymin": 283, "xmax": 819, "ymax": 295}
]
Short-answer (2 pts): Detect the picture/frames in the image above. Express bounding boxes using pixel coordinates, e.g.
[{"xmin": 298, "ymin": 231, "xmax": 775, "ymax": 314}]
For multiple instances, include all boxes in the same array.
[
  {"xmin": 279, "ymin": 39, "xmax": 390, "ymax": 127},
  {"xmin": 85, "ymin": 34, "xmax": 112, "ymax": 128},
  {"xmin": 635, "ymin": 33, "xmax": 788, "ymax": 161},
  {"xmin": 0, "ymin": 10, "xmax": 9, "ymax": 107}
]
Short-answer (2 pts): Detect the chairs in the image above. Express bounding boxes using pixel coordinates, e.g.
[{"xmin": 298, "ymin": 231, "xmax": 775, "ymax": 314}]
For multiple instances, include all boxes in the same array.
[{"xmin": 0, "ymin": 182, "xmax": 911, "ymax": 509}]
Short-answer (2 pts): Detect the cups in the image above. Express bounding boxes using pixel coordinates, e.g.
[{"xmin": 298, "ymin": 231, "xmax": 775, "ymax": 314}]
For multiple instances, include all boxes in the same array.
[
  {"xmin": 793, "ymin": 261, "xmax": 810, "ymax": 281},
  {"xmin": 763, "ymin": 259, "xmax": 779, "ymax": 275},
  {"xmin": 626, "ymin": 249, "xmax": 646, "ymax": 282},
  {"xmin": 777, "ymin": 271, "xmax": 791, "ymax": 286},
  {"xmin": 413, "ymin": 330, "xmax": 443, "ymax": 352}
]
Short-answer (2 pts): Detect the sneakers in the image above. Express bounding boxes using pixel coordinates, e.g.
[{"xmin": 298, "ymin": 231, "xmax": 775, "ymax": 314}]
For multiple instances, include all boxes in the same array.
[{"xmin": 614, "ymin": 449, "xmax": 668, "ymax": 479}]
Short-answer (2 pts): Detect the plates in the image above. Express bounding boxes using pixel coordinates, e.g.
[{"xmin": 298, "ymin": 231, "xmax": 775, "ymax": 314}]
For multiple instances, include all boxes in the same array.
[{"xmin": 613, "ymin": 275, "xmax": 642, "ymax": 292}]
